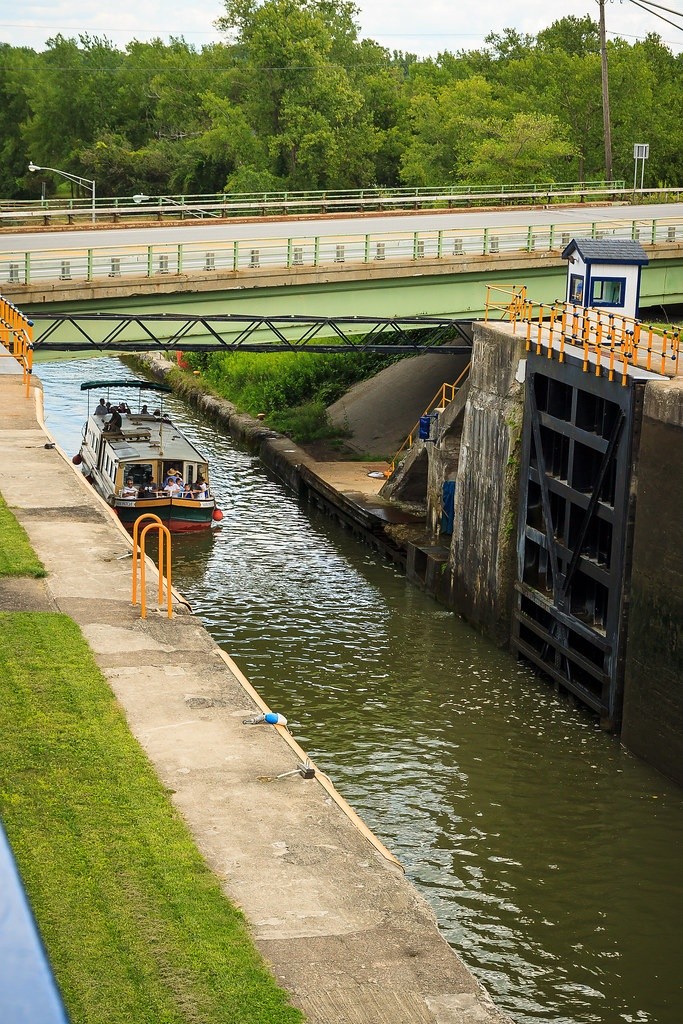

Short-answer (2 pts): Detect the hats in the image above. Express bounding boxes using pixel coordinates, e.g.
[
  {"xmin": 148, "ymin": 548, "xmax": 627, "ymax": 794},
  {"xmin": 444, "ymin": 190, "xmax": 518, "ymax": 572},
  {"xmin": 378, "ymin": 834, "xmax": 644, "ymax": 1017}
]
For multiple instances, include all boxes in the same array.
[
  {"xmin": 167, "ymin": 468, "xmax": 176, "ymax": 476},
  {"xmin": 176, "ymin": 471, "xmax": 182, "ymax": 477}
]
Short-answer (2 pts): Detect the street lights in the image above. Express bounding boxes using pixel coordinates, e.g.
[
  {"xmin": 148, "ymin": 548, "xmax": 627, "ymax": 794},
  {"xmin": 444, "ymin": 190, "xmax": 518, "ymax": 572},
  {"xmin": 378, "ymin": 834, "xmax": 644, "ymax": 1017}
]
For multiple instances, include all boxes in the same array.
[{"xmin": 28, "ymin": 164, "xmax": 95, "ymax": 223}]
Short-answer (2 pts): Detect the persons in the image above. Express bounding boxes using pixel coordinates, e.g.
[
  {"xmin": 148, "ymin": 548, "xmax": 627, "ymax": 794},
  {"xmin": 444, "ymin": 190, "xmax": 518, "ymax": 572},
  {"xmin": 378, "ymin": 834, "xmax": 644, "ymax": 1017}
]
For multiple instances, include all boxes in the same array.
[
  {"xmin": 154, "ymin": 408, "xmax": 160, "ymax": 416},
  {"xmin": 95, "ymin": 398, "xmax": 131, "ymax": 414},
  {"xmin": 141, "ymin": 405, "xmax": 149, "ymax": 414},
  {"xmin": 122, "ymin": 475, "xmax": 165, "ymax": 499},
  {"xmin": 161, "ymin": 468, "xmax": 209, "ymax": 500},
  {"xmin": 107, "ymin": 408, "xmax": 122, "ymax": 431}
]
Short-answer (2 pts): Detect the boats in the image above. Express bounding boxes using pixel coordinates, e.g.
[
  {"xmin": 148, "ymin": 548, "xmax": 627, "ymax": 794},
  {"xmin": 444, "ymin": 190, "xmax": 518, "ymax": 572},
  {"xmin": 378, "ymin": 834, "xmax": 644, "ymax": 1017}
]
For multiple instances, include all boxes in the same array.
[{"xmin": 72, "ymin": 379, "xmax": 223, "ymax": 533}]
